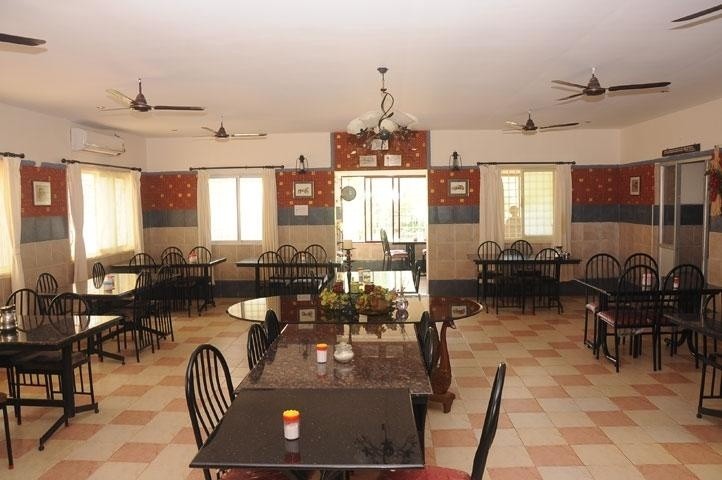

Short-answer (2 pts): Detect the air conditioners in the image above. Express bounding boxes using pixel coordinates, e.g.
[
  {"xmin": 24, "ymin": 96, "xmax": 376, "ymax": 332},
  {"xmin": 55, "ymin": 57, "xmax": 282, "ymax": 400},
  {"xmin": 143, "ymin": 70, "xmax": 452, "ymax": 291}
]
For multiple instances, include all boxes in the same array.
[{"xmin": 69, "ymin": 127, "xmax": 126, "ymax": 157}]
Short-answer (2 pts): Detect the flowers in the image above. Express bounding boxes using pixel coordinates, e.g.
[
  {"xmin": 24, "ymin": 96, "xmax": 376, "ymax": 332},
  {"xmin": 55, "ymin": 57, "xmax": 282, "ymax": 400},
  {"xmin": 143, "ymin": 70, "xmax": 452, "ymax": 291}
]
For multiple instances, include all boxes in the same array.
[{"xmin": 320, "ymin": 285, "xmax": 388, "ymax": 324}]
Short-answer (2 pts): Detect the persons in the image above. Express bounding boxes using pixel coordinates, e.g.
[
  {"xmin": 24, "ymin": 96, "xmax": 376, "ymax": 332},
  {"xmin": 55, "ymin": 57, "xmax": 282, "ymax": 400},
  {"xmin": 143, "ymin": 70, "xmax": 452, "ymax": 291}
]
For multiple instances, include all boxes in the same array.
[{"xmin": 505, "ymin": 206, "xmax": 522, "ymax": 236}]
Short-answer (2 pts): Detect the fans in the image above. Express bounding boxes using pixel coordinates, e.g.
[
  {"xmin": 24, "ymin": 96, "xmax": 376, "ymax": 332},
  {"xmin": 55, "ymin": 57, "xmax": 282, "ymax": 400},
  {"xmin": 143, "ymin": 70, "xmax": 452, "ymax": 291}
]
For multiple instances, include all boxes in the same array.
[
  {"xmin": 341, "ymin": 186, "xmax": 356, "ymax": 201},
  {"xmin": 502, "ymin": 108, "xmax": 578, "ymax": 135},
  {"xmin": 551, "ymin": 67, "xmax": 671, "ymax": 102},
  {"xmin": 96, "ymin": 77, "xmax": 205, "ymax": 113},
  {"xmin": 671, "ymin": 3, "xmax": 722, "ymax": 23},
  {"xmin": 193, "ymin": 113, "xmax": 267, "ymax": 142},
  {"xmin": 0, "ymin": 33, "xmax": 46, "ymax": 46}
]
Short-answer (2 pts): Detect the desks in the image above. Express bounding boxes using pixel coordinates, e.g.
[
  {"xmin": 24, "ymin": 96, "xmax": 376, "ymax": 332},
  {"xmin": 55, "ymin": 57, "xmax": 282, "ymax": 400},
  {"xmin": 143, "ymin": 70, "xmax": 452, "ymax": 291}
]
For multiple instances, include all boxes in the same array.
[
  {"xmin": 233, "ymin": 322, "xmax": 430, "ymax": 449},
  {"xmin": 187, "ymin": 388, "xmax": 425, "ymax": 480},
  {"xmin": 317, "ymin": 270, "xmax": 416, "ymax": 292},
  {"xmin": 233, "ymin": 257, "xmax": 343, "ymax": 298},
  {"xmin": 37, "ymin": 272, "xmax": 180, "ymax": 364},
  {"xmin": 471, "ymin": 255, "xmax": 583, "ymax": 313},
  {"xmin": 391, "ymin": 237, "xmax": 428, "ymax": 285},
  {"xmin": 0, "ymin": 312, "xmax": 124, "ymax": 450},
  {"xmin": 110, "ymin": 256, "xmax": 226, "ymax": 317},
  {"xmin": 576, "ymin": 275, "xmax": 721, "ymax": 365},
  {"xmin": 227, "ymin": 292, "xmax": 484, "ymax": 414}
]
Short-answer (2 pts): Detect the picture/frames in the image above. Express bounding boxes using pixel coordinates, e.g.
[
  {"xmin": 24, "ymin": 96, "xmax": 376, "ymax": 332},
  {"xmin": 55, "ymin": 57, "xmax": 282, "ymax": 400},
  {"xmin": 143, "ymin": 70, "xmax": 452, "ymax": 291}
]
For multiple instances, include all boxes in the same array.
[
  {"xmin": 447, "ymin": 178, "xmax": 469, "ymax": 198},
  {"xmin": 292, "ymin": 181, "xmax": 314, "ymax": 199},
  {"xmin": 32, "ymin": 181, "xmax": 51, "ymax": 206},
  {"xmin": 629, "ymin": 176, "xmax": 640, "ymax": 196}
]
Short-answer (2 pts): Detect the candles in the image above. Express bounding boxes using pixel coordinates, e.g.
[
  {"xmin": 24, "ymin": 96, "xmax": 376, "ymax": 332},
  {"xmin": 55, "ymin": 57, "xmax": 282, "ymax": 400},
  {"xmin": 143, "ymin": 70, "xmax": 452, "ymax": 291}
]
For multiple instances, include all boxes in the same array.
[{"xmin": 343, "ymin": 238, "xmax": 351, "ymax": 249}]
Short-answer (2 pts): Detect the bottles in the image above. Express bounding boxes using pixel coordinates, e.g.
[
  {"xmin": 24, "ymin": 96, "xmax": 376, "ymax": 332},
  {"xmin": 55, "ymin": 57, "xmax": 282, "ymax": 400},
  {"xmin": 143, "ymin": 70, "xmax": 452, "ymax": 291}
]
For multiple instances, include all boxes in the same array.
[
  {"xmin": 316, "ymin": 343, "xmax": 328, "ymax": 363},
  {"xmin": 317, "ymin": 363, "xmax": 328, "ymax": 379},
  {"xmin": 673, "ymin": 288, "xmax": 679, "ymax": 290},
  {"xmin": 674, "ymin": 276, "xmax": 679, "ymax": 288},
  {"xmin": 188, "ymin": 253, "xmax": 198, "ymax": 263},
  {"xmin": 284, "ymin": 441, "xmax": 300, "ymax": 463},
  {"xmin": 282, "ymin": 409, "xmax": 301, "ymax": 440},
  {"xmin": 105, "ymin": 275, "xmax": 115, "ymax": 291}
]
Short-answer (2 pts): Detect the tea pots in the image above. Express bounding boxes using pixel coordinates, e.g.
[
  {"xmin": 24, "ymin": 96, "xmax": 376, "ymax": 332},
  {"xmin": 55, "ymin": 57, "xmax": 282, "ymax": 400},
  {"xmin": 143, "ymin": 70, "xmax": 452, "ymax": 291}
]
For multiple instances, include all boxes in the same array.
[
  {"xmin": 553, "ymin": 245, "xmax": 568, "ymax": 258},
  {"xmin": 334, "ymin": 341, "xmax": 354, "ymax": 364},
  {"xmin": 1, "ymin": 304, "xmax": 18, "ymax": 333},
  {"xmin": 336, "ymin": 365, "xmax": 356, "ymax": 382},
  {"xmin": 2, "ymin": 332, "xmax": 19, "ymax": 343}
]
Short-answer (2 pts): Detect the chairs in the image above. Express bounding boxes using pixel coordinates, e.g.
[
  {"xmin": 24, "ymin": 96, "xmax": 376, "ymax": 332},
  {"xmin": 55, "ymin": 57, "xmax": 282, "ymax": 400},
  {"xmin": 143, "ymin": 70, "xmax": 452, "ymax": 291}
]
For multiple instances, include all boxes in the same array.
[
  {"xmin": 416, "ymin": 311, "xmax": 438, "ymax": 374},
  {"xmin": 256, "ymin": 244, "xmax": 327, "ymax": 299},
  {"xmin": 380, "ymin": 228, "xmax": 409, "ymax": 272},
  {"xmin": 378, "ymin": 363, "xmax": 507, "ymax": 480},
  {"xmin": 91, "ymin": 247, "xmax": 215, "ymax": 318},
  {"xmin": 475, "ymin": 240, "xmax": 561, "ymax": 315},
  {"xmin": 248, "ymin": 310, "xmax": 279, "ymax": 372},
  {"xmin": 584, "ymin": 253, "xmax": 704, "ymax": 374},
  {"xmin": 35, "ymin": 273, "xmax": 71, "ymax": 314},
  {"xmin": 99, "ymin": 264, "xmax": 174, "ymax": 363},
  {"xmin": 184, "ymin": 343, "xmax": 321, "ymax": 480},
  {"xmin": 5, "ymin": 289, "xmax": 95, "ymax": 426},
  {"xmin": 414, "ymin": 248, "xmax": 427, "ymax": 294},
  {"xmin": 0, "ymin": 392, "xmax": 13, "ymax": 468},
  {"xmin": 695, "ymin": 289, "xmax": 720, "ymax": 418}
]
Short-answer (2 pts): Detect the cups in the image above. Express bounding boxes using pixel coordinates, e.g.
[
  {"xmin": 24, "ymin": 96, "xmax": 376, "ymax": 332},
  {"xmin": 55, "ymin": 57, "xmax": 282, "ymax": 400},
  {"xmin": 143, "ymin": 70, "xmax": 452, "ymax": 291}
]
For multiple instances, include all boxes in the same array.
[
  {"xmin": 642, "ymin": 274, "xmax": 654, "ymax": 286},
  {"xmin": 640, "ymin": 286, "xmax": 652, "ymax": 291},
  {"xmin": 396, "ymin": 300, "xmax": 407, "ymax": 311},
  {"xmin": 396, "ymin": 310, "xmax": 409, "ymax": 321}
]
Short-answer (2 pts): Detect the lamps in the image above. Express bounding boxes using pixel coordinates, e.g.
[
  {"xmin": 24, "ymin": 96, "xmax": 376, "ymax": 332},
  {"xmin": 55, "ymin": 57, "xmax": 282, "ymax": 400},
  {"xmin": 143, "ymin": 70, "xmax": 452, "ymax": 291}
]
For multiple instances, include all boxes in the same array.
[
  {"xmin": 346, "ymin": 67, "xmax": 417, "ymax": 145},
  {"xmin": 295, "ymin": 155, "xmax": 308, "ymax": 173},
  {"xmin": 448, "ymin": 152, "xmax": 463, "ymax": 171}
]
